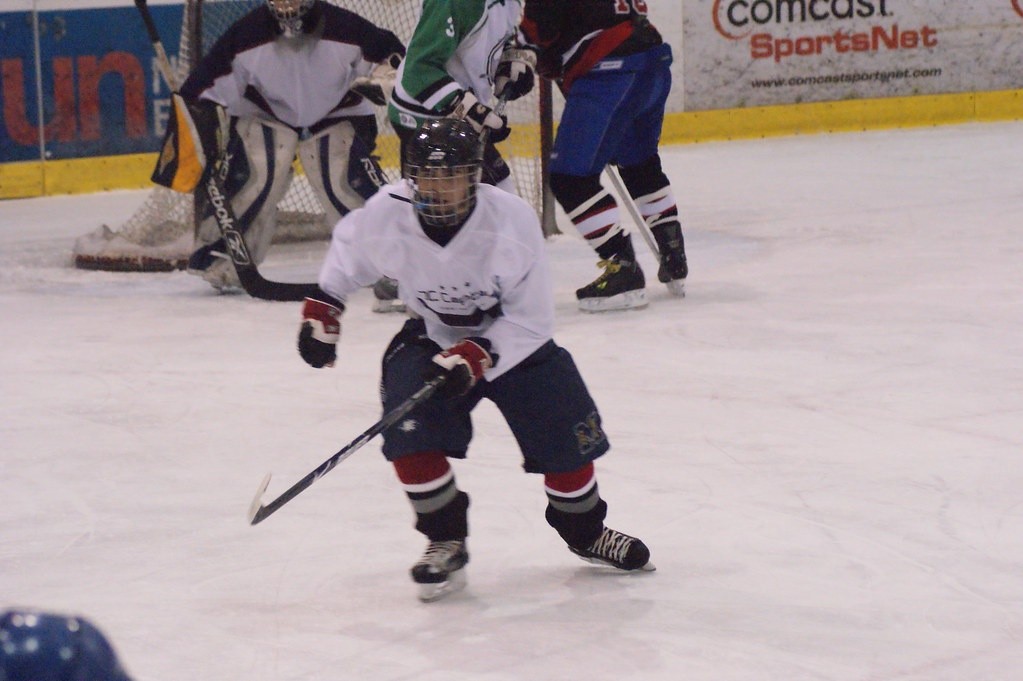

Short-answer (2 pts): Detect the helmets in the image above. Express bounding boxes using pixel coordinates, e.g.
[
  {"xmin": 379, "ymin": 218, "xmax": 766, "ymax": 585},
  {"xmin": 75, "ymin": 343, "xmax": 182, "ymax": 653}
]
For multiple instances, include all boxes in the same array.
[
  {"xmin": 0, "ymin": 611, "xmax": 131, "ymax": 681},
  {"xmin": 404, "ymin": 119, "xmax": 479, "ymax": 228}
]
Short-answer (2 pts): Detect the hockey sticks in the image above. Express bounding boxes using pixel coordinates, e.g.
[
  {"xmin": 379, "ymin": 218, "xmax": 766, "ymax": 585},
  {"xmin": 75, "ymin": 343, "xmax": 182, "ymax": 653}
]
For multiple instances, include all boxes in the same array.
[
  {"xmin": 137, "ymin": 2, "xmax": 352, "ymax": 303},
  {"xmin": 247, "ymin": 365, "xmax": 459, "ymax": 524},
  {"xmin": 553, "ymin": 75, "xmax": 663, "ymax": 265},
  {"xmin": 477, "ymin": 79, "xmax": 517, "ymax": 186}
]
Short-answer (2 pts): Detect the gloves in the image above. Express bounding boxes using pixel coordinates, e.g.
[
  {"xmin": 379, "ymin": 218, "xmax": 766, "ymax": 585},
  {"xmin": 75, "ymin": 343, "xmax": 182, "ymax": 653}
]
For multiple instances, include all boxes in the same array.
[
  {"xmin": 425, "ymin": 336, "xmax": 500, "ymax": 401},
  {"xmin": 299, "ymin": 286, "xmax": 346, "ymax": 368},
  {"xmin": 493, "ymin": 43, "xmax": 536, "ymax": 100},
  {"xmin": 453, "ymin": 87, "xmax": 511, "ymax": 143}
]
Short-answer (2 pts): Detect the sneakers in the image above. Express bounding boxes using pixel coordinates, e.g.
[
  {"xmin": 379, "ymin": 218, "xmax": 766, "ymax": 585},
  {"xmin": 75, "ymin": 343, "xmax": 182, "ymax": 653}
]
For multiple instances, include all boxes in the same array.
[
  {"xmin": 657, "ymin": 223, "xmax": 688, "ymax": 298},
  {"xmin": 411, "ymin": 538, "xmax": 470, "ymax": 603},
  {"xmin": 576, "ymin": 233, "xmax": 648, "ymax": 312},
  {"xmin": 569, "ymin": 525, "xmax": 657, "ymax": 571}
]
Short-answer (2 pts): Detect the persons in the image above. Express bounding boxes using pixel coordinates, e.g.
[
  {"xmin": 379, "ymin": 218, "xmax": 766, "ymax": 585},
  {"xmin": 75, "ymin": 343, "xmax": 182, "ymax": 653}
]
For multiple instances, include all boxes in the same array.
[
  {"xmin": 373, "ymin": 0, "xmax": 537, "ymax": 313},
  {"xmin": 518, "ymin": 0, "xmax": 688, "ymax": 310},
  {"xmin": 298, "ymin": 118, "xmax": 655, "ymax": 602},
  {"xmin": 0, "ymin": 610, "xmax": 126, "ymax": 681},
  {"xmin": 152, "ymin": 0, "xmax": 407, "ymax": 291}
]
